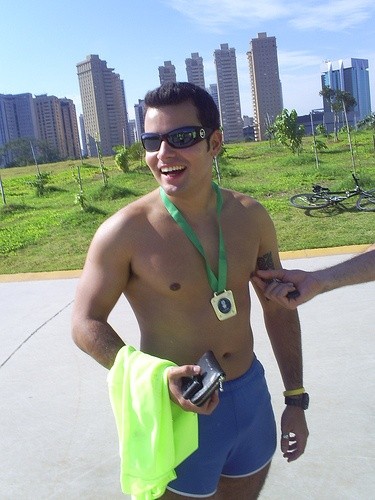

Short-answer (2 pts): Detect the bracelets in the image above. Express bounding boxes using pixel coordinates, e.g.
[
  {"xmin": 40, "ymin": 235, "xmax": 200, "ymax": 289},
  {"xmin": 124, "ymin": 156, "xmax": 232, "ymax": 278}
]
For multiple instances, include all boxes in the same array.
[{"xmin": 283, "ymin": 387, "xmax": 305, "ymax": 397}]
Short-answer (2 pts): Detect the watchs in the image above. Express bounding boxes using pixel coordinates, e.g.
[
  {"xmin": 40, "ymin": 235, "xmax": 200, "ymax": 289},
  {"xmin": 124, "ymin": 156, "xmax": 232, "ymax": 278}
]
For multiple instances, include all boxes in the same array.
[{"xmin": 285, "ymin": 393, "xmax": 309, "ymax": 410}]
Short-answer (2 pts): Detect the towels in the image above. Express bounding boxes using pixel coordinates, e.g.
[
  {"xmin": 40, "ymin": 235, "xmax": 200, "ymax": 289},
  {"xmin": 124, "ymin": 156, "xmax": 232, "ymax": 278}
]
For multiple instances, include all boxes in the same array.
[{"xmin": 107, "ymin": 345, "xmax": 199, "ymax": 500}]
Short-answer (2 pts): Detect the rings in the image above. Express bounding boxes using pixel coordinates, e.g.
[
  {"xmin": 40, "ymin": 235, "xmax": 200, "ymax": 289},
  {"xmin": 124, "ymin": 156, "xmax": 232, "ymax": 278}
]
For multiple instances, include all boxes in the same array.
[{"xmin": 281, "ymin": 435, "xmax": 290, "ymax": 440}]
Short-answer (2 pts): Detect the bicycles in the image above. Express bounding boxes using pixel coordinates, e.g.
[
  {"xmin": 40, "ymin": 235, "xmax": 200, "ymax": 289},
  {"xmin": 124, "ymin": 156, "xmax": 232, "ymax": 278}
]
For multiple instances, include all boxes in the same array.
[{"xmin": 289, "ymin": 173, "xmax": 375, "ymax": 212}]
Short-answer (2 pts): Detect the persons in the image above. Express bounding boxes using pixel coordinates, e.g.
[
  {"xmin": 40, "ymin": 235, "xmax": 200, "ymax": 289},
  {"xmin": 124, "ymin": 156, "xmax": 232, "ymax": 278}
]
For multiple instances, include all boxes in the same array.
[
  {"xmin": 71, "ymin": 81, "xmax": 309, "ymax": 500},
  {"xmin": 253, "ymin": 249, "xmax": 375, "ymax": 310}
]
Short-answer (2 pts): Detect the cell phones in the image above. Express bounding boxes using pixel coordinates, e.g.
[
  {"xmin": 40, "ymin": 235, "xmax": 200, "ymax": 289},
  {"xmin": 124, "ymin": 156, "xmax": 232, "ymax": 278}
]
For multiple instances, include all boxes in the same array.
[{"xmin": 180, "ymin": 374, "xmax": 202, "ymax": 400}]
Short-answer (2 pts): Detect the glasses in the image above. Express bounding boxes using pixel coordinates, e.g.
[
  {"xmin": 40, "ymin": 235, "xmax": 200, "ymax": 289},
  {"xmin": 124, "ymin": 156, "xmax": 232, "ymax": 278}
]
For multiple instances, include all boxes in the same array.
[{"xmin": 140, "ymin": 126, "xmax": 216, "ymax": 152}]
